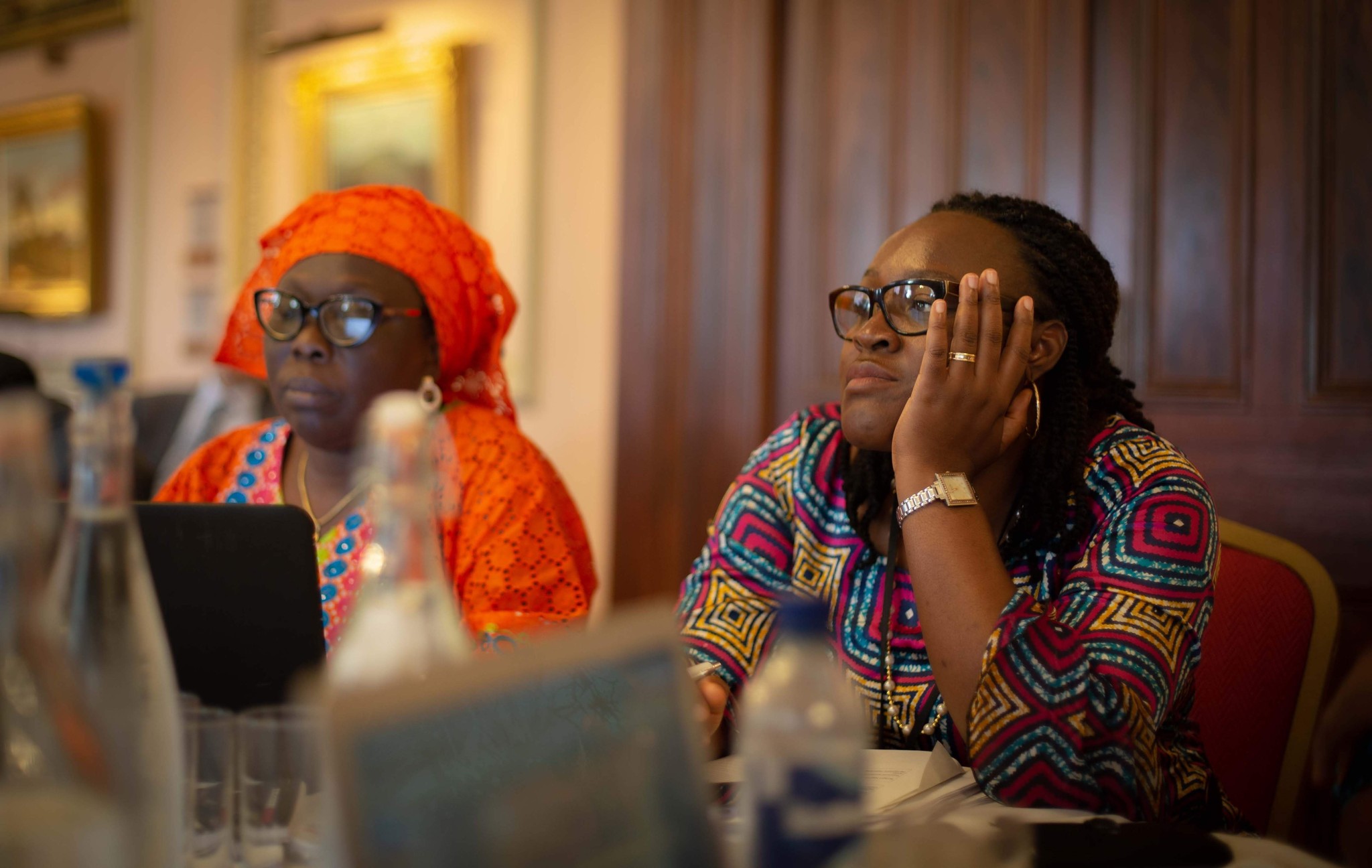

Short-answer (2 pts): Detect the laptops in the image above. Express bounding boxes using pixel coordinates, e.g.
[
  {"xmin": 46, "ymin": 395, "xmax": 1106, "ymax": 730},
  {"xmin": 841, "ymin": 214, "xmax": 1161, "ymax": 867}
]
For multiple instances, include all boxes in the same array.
[
  {"xmin": 318, "ymin": 595, "xmax": 721, "ymax": 868},
  {"xmin": 125, "ymin": 500, "xmax": 323, "ymax": 715}
]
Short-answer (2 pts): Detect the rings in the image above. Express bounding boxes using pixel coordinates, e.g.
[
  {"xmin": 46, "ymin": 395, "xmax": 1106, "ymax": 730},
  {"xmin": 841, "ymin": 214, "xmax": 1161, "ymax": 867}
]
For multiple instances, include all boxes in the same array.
[{"xmin": 948, "ymin": 352, "xmax": 976, "ymax": 363}]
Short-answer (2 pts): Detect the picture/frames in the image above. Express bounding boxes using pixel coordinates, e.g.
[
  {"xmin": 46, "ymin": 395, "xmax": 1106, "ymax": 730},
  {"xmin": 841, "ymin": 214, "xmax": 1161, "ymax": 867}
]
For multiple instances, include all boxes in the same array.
[
  {"xmin": 0, "ymin": 94, "xmax": 95, "ymax": 320},
  {"xmin": 294, "ymin": 44, "xmax": 460, "ymax": 219}
]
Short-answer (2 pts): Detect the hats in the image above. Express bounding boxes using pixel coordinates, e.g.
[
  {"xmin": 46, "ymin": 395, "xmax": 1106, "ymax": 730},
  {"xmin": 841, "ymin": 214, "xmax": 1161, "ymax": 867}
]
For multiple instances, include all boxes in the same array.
[{"xmin": 214, "ymin": 184, "xmax": 520, "ymax": 426}]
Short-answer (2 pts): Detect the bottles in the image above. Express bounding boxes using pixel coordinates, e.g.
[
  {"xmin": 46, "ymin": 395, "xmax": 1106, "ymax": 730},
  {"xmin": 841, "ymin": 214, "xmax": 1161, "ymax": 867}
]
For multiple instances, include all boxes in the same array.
[
  {"xmin": 36, "ymin": 356, "xmax": 187, "ymax": 868},
  {"xmin": 320, "ymin": 380, "xmax": 466, "ymax": 868},
  {"xmin": 0, "ymin": 397, "xmax": 55, "ymax": 868},
  {"xmin": 739, "ymin": 597, "xmax": 866, "ymax": 868}
]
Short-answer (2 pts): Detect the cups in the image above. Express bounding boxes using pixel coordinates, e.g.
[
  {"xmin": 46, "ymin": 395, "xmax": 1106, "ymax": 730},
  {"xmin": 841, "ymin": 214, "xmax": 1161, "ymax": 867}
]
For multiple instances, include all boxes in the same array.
[
  {"xmin": 184, "ymin": 709, "xmax": 238, "ymax": 867},
  {"xmin": 240, "ymin": 706, "xmax": 320, "ymax": 868}
]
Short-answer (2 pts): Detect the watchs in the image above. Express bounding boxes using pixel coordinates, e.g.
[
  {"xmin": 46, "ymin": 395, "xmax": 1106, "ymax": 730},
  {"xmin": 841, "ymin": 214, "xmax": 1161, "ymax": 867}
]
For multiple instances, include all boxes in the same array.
[{"xmin": 895, "ymin": 471, "xmax": 981, "ymax": 529}]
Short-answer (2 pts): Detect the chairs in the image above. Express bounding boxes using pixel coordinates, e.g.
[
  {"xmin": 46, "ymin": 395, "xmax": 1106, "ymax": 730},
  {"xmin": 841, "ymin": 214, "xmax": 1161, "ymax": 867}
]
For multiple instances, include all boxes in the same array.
[{"xmin": 1190, "ymin": 518, "xmax": 1336, "ymax": 845}]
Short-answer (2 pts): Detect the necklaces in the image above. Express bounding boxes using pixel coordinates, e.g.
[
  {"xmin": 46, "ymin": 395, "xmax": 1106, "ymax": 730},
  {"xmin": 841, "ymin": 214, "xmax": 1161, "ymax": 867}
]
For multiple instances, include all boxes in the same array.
[
  {"xmin": 884, "ymin": 575, "xmax": 949, "ymax": 737},
  {"xmin": 296, "ymin": 450, "xmax": 362, "ymax": 541}
]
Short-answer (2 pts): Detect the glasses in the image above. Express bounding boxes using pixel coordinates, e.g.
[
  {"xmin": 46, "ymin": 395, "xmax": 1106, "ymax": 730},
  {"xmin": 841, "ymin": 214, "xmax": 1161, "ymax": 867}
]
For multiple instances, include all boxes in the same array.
[
  {"xmin": 828, "ymin": 278, "xmax": 1049, "ymax": 343},
  {"xmin": 254, "ymin": 287, "xmax": 430, "ymax": 349}
]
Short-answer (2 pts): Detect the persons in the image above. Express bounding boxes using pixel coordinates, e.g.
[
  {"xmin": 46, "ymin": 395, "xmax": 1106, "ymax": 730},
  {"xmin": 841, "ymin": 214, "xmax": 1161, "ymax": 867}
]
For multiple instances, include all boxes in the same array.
[
  {"xmin": 153, "ymin": 182, "xmax": 600, "ymax": 657},
  {"xmin": 675, "ymin": 190, "xmax": 1245, "ymax": 838}
]
